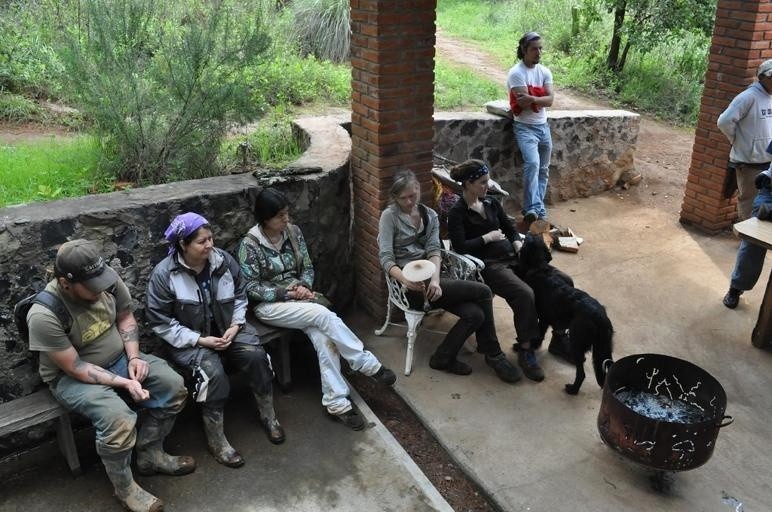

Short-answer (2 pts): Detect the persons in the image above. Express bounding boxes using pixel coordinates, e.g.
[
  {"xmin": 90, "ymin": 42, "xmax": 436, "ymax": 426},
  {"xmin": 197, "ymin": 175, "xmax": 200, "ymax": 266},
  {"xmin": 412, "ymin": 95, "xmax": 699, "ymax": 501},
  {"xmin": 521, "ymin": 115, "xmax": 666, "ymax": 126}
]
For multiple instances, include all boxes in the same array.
[
  {"xmin": 238, "ymin": 184, "xmax": 396, "ymax": 429},
  {"xmin": 508, "ymin": 31, "xmax": 554, "ymax": 225},
  {"xmin": 144, "ymin": 210, "xmax": 284, "ymax": 467},
  {"xmin": 26, "ymin": 239, "xmax": 197, "ymax": 511},
  {"xmin": 379, "ymin": 169, "xmax": 525, "ymax": 383},
  {"xmin": 447, "ymin": 158, "xmax": 587, "ymax": 382},
  {"xmin": 716, "ymin": 59, "xmax": 772, "ymax": 223},
  {"xmin": 723, "ymin": 138, "xmax": 772, "ymax": 308}
]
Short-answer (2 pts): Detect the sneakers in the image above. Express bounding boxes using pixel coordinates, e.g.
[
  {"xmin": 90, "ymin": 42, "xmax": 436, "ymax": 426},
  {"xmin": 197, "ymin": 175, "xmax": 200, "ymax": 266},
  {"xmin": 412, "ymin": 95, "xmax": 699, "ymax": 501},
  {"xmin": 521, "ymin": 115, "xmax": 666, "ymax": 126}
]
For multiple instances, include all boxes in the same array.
[
  {"xmin": 522, "ymin": 211, "xmax": 538, "ymax": 223},
  {"xmin": 549, "ymin": 342, "xmax": 586, "ymax": 364},
  {"xmin": 430, "ymin": 356, "xmax": 472, "ymax": 376},
  {"xmin": 518, "ymin": 349, "xmax": 544, "ymax": 381},
  {"xmin": 723, "ymin": 285, "xmax": 744, "ymax": 309},
  {"xmin": 336, "ymin": 410, "xmax": 365, "ymax": 431},
  {"xmin": 370, "ymin": 365, "xmax": 397, "ymax": 387},
  {"xmin": 485, "ymin": 352, "xmax": 521, "ymax": 383}
]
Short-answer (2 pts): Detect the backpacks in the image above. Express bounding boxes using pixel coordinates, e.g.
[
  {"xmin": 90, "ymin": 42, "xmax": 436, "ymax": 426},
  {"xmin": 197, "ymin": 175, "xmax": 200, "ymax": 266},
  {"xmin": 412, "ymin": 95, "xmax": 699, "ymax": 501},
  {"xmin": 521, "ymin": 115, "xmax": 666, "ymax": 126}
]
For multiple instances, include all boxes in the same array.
[{"xmin": 13, "ymin": 289, "xmax": 69, "ymax": 373}]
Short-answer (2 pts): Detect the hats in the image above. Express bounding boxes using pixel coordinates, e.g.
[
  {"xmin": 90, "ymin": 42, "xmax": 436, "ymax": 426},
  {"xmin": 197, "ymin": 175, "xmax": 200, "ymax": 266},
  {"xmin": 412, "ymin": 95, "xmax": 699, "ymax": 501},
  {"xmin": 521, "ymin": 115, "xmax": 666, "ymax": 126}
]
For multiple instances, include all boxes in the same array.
[
  {"xmin": 55, "ymin": 239, "xmax": 118, "ymax": 294},
  {"xmin": 757, "ymin": 59, "xmax": 772, "ymax": 80}
]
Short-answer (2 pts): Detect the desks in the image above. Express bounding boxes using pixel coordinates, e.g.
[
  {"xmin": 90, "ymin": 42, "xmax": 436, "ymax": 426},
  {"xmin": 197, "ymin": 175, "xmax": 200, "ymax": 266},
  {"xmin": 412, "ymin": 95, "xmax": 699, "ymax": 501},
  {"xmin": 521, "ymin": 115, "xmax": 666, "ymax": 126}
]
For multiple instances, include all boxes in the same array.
[{"xmin": 733, "ymin": 217, "xmax": 771, "ymax": 348}]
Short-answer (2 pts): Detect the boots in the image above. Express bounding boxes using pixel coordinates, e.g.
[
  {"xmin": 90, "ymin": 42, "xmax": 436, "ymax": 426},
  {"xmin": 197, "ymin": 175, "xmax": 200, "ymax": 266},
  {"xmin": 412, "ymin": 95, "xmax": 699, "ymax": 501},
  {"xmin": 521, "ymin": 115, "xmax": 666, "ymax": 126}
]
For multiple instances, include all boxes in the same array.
[
  {"xmin": 201, "ymin": 405, "xmax": 246, "ymax": 469},
  {"xmin": 249, "ymin": 380, "xmax": 286, "ymax": 445},
  {"xmin": 100, "ymin": 448, "xmax": 164, "ymax": 512},
  {"xmin": 134, "ymin": 411, "xmax": 197, "ymax": 476}
]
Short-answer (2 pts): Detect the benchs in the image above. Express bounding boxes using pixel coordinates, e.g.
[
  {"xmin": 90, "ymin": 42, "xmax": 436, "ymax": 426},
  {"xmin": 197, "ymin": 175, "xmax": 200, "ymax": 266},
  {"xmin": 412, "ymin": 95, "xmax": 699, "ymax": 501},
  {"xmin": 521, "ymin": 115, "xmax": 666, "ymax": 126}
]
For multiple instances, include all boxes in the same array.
[{"xmin": 0, "ymin": 296, "xmax": 331, "ymax": 478}]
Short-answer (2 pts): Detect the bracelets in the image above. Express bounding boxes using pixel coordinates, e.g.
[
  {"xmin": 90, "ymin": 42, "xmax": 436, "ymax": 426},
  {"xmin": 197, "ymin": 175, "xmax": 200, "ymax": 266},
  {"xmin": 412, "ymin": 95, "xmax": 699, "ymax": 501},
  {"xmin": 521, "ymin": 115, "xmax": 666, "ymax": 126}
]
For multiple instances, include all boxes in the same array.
[{"xmin": 127, "ymin": 357, "xmax": 142, "ymax": 363}]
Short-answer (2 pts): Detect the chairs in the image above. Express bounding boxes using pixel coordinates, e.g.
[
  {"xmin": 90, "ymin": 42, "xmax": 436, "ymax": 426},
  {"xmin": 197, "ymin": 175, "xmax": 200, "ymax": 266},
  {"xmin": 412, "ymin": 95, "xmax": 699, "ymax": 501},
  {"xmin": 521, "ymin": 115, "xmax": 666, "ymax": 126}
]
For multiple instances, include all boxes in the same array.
[
  {"xmin": 433, "ymin": 152, "xmax": 509, "ymax": 210},
  {"xmin": 442, "ymin": 233, "xmax": 526, "ymax": 283},
  {"xmin": 375, "ymin": 233, "xmax": 476, "ymax": 376}
]
